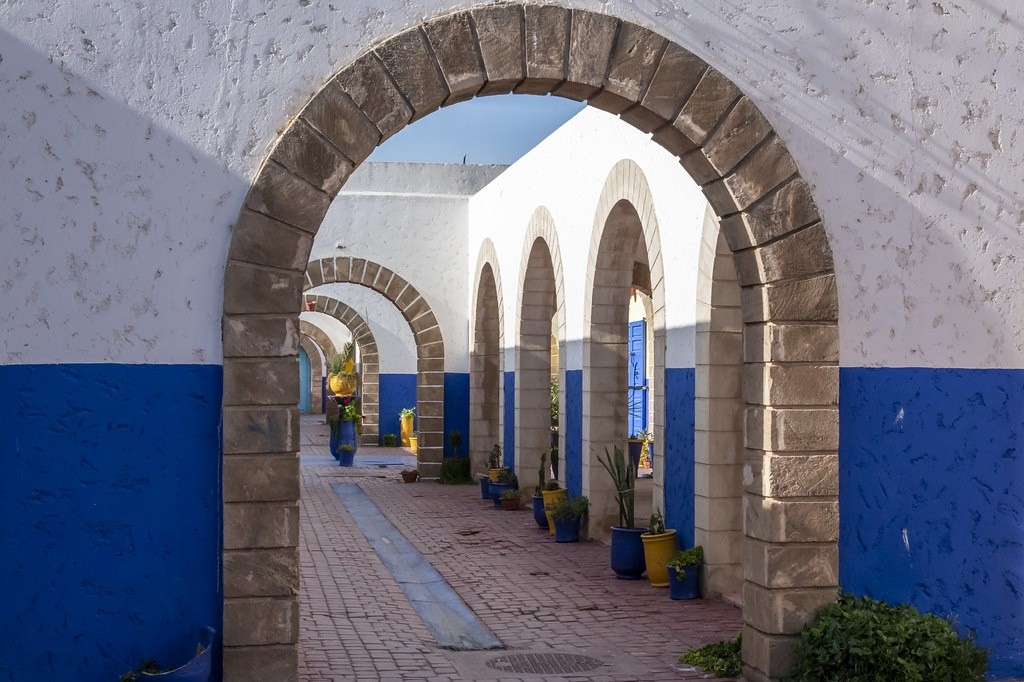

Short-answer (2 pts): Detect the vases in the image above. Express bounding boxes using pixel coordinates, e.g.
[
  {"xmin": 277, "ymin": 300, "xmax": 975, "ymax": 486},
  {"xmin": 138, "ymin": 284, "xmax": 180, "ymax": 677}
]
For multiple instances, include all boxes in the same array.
[
  {"xmin": 401, "ymin": 470, "xmax": 417, "ymax": 483},
  {"xmin": 629, "ymin": 442, "xmax": 642, "ymax": 478},
  {"xmin": 480, "ymin": 476, "xmax": 491, "ymax": 499},
  {"xmin": 648, "ymin": 444, "xmax": 654, "ymax": 470},
  {"xmin": 409, "ymin": 436, "xmax": 417, "ymax": 454},
  {"xmin": 329, "ymin": 420, "xmax": 357, "ymax": 460}
]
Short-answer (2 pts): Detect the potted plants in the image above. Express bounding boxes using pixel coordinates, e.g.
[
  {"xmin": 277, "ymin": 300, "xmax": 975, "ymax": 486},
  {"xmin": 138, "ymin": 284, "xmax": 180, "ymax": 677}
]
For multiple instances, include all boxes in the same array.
[
  {"xmin": 667, "ymin": 545, "xmax": 703, "ymax": 600},
  {"xmin": 500, "ymin": 488, "xmax": 522, "ymax": 510},
  {"xmin": 492, "ymin": 471, "xmax": 518, "ymax": 508},
  {"xmin": 532, "ymin": 453, "xmax": 549, "ymax": 529},
  {"xmin": 338, "ymin": 443, "xmax": 355, "ymax": 466},
  {"xmin": 551, "ymin": 495, "xmax": 591, "ymax": 542},
  {"xmin": 324, "ymin": 342, "xmax": 359, "ymax": 397},
  {"xmin": 640, "ymin": 507, "xmax": 678, "ymax": 588},
  {"xmin": 486, "ymin": 444, "xmax": 510, "ymax": 482},
  {"xmin": 399, "ymin": 406, "xmax": 416, "ymax": 447},
  {"xmin": 590, "ymin": 444, "xmax": 650, "ymax": 579},
  {"xmin": 542, "ymin": 479, "xmax": 569, "ymax": 535}
]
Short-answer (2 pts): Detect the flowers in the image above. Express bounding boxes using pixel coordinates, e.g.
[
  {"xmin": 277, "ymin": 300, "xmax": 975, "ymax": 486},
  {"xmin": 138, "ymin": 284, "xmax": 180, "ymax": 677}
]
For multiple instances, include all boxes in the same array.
[{"xmin": 326, "ymin": 399, "xmax": 366, "ymax": 439}]
